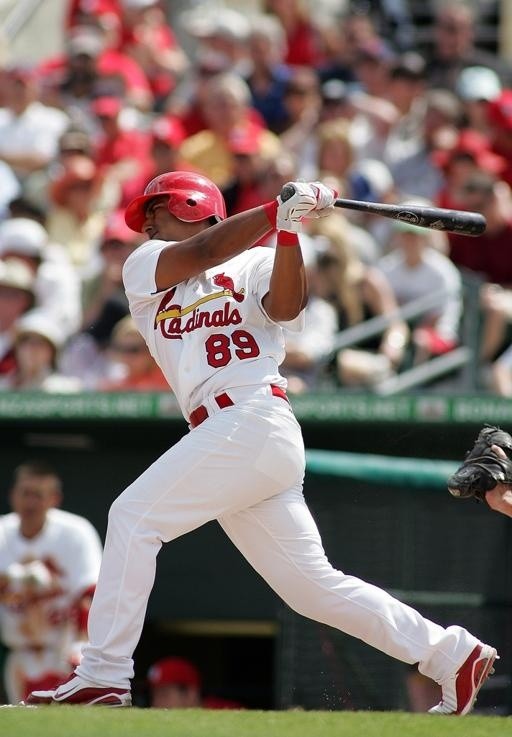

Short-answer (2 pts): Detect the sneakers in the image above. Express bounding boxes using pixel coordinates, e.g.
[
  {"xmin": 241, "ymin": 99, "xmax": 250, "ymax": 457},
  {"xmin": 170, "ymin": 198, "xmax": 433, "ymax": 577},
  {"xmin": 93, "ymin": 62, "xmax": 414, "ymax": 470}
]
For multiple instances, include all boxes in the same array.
[
  {"xmin": 19, "ymin": 673, "xmax": 133, "ymax": 708},
  {"xmin": 428, "ymin": 638, "xmax": 499, "ymax": 716}
]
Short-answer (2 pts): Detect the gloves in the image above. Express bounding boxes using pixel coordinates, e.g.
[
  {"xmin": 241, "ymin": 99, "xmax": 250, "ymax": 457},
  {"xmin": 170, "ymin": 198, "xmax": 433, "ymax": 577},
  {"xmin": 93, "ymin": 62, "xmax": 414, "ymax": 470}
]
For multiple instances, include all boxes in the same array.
[{"xmin": 272, "ymin": 181, "xmax": 339, "ymax": 235}]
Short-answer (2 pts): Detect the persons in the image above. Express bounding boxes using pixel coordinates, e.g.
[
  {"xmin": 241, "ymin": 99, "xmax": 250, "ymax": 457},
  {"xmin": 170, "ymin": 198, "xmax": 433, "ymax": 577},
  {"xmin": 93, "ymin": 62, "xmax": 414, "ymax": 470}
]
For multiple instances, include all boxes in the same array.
[
  {"xmin": 25, "ymin": 171, "xmax": 500, "ymax": 715},
  {"xmin": 0, "ymin": 462, "xmax": 103, "ymax": 704},
  {"xmin": 1, "ymin": 0, "xmax": 511, "ymax": 395},
  {"xmin": 474, "ymin": 444, "xmax": 512, "ymax": 519},
  {"xmin": 147, "ymin": 658, "xmax": 241, "ymax": 710}
]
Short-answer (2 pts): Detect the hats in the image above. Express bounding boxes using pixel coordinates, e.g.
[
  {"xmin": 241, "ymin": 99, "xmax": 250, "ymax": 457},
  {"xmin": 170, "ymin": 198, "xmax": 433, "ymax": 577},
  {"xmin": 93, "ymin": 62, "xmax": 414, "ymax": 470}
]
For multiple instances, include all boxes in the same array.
[
  {"xmin": 147, "ymin": 657, "xmax": 199, "ymax": 689},
  {"xmin": 394, "ymin": 200, "xmax": 432, "ymax": 237},
  {"xmin": 455, "ymin": 65, "xmax": 503, "ymax": 105},
  {"xmin": 1, "ymin": 219, "xmax": 63, "ymax": 355},
  {"xmin": 448, "ymin": 128, "xmax": 490, "ymax": 168},
  {"xmin": 341, "ymin": 158, "xmax": 396, "ymax": 203}
]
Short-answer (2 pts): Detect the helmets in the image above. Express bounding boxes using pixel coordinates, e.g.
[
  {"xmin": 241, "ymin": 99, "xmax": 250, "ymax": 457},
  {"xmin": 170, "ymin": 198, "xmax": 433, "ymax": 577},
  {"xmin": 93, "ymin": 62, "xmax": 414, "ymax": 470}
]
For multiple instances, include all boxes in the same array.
[{"xmin": 124, "ymin": 171, "xmax": 228, "ymax": 233}]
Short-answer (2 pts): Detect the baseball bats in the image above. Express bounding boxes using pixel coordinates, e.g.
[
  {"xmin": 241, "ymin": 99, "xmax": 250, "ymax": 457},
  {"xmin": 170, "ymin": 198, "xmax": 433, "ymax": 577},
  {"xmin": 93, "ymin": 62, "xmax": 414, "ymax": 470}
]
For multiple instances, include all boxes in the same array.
[{"xmin": 280, "ymin": 184, "xmax": 486, "ymax": 239}]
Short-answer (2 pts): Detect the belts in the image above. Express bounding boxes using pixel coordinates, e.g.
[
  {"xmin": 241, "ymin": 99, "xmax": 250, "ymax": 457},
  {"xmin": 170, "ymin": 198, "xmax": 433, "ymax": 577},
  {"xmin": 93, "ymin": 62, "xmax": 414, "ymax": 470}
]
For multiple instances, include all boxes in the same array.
[{"xmin": 189, "ymin": 385, "xmax": 288, "ymax": 430}]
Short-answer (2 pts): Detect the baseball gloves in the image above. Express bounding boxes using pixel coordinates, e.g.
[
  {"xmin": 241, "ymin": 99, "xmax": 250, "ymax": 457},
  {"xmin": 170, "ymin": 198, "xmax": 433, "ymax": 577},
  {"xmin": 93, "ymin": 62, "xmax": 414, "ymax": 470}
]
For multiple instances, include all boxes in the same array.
[{"xmin": 448, "ymin": 424, "xmax": 511, "ymax": 505}]
